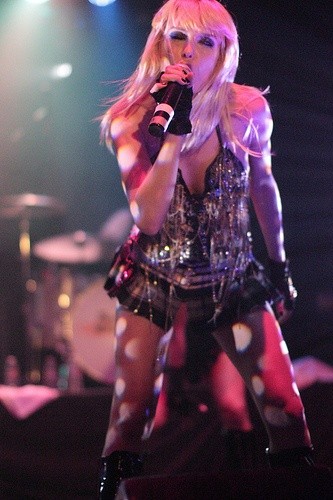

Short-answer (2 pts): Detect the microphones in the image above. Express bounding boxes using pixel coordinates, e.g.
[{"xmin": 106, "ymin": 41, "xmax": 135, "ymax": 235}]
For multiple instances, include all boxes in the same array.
[{"xmin": 148, "ymin": 82, "xmax": 185, "ymax": 138}]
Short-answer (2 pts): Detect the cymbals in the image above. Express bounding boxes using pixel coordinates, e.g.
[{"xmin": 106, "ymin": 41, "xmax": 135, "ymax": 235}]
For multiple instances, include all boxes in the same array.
[
  {"xmin": 34, "ymin": 235, "xmax": 113, "ymax": 262},
  {"xmin": 1, "ymin": 192, "xmax": 68, "ymax": 219}
]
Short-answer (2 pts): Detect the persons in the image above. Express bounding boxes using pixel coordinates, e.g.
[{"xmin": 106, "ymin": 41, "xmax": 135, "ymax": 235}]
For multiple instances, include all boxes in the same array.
[{"xmin": 93, "ymin": 0, "xmax": 321, "ymax": 500}]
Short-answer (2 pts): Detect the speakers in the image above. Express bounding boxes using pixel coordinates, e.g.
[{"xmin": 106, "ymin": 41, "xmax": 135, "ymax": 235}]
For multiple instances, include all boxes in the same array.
[{"xmin": 116, "ymin": 464, "xmax": 333, "ymax": 500}]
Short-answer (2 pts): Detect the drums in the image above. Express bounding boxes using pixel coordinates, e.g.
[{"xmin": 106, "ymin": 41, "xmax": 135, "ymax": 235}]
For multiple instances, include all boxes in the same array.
[{"xmin": 70, "ymin": 274, "xmax": 134, "ymax": 387}]
